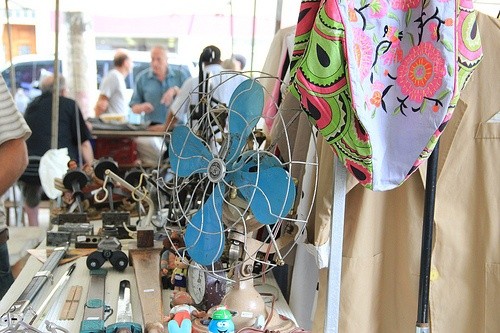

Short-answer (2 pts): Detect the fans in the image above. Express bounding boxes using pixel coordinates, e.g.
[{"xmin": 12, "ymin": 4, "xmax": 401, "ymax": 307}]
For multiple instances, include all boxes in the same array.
[{"xmin": 156, "ymin": 70, "xmax": 319, "ymax": 328}]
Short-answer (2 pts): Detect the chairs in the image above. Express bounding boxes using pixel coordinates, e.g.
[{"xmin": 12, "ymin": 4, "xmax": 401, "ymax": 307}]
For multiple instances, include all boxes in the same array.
[{"xmin": 12, "ymin": 155, "xmax": 58, "ymax": 229}]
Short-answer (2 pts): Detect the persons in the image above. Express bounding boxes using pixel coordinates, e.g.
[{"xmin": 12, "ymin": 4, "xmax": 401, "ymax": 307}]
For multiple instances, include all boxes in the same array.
[
  {"xmin": 170, "ymin": 250, "xmax": 190, "ymax": 292},
  {"xmin": 21, "ymin": 75, "xmax": 95, "ymax": 226},
  {"xmin": 129, "ymin": 45, "xmax": 251, "ymax": 156},
  {"xmin": 93, "ymin": 50, "xmax": 132, "ymax": 120},
  {"xmin": 159, "ymin": 231, "xmax": 186, "ymax": 290},
  {"xmin": 0, "ymin": 73, "xmax": 32, "ymax": 301},
  {"xmin": 164, "ymin": 292, "xmax": 205, "ymax": 333}
]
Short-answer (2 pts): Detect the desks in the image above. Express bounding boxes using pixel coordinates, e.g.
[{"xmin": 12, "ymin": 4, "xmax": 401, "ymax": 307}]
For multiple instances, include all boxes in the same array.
[
  {"xmin": 85, "ymin": 125, "xmax": 175, "ymax": 138},
  {"xmin": 0, "ymin": 198, "xmax": 304, "ymax": 333}
]
